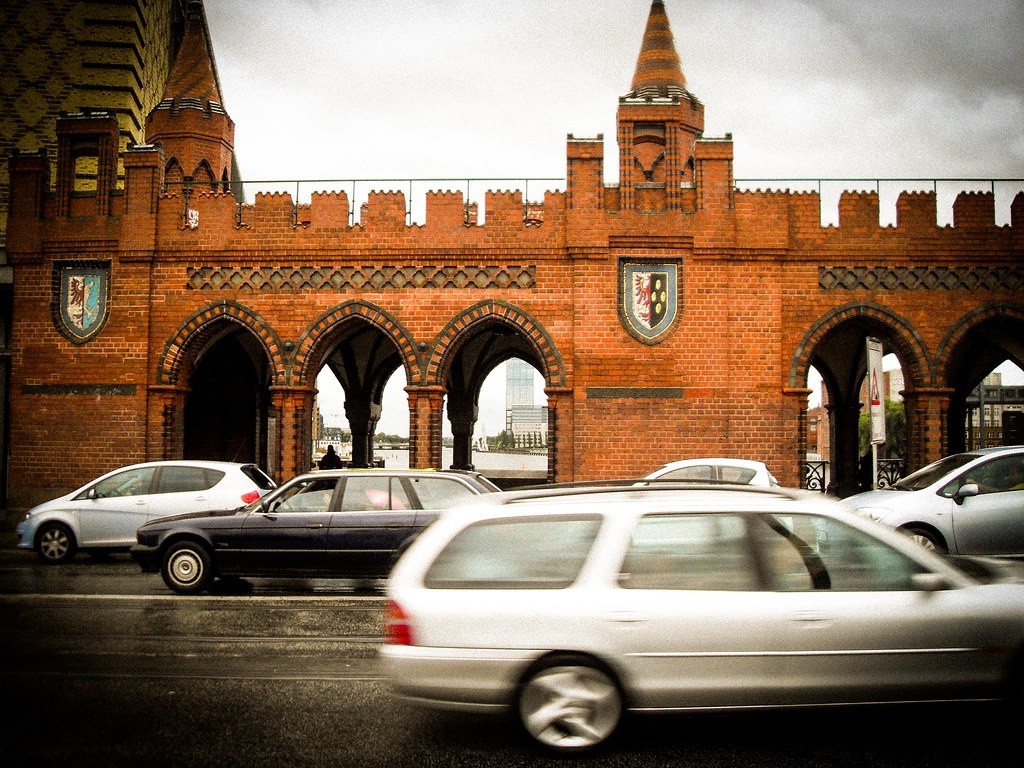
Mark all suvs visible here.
[378,479,1024,759]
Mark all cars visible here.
[815,444,1024,569]
[630,458,779,492]
[132,468,503,595]
[17,460,281,563]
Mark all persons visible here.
[966,462,1024,493]
[319,445,342,470]
